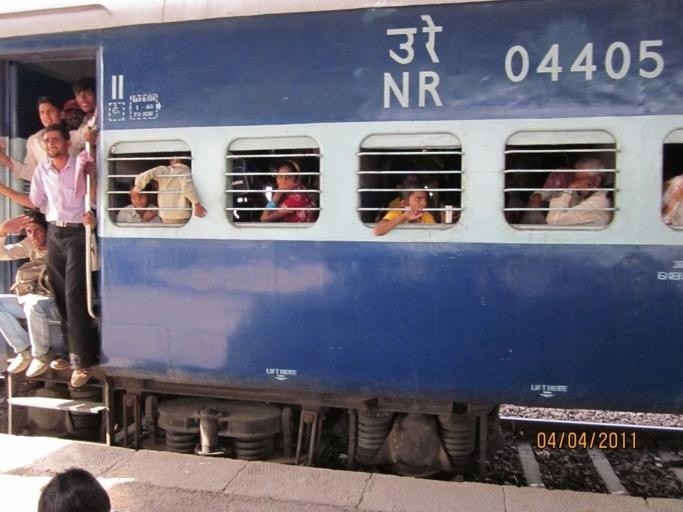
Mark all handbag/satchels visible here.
[15,261,53,297]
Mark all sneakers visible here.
[49,357,69,371]
[26,348,55,378]
[70,366,95,389]
[7,347,32,375]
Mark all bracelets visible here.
[265,201,277,211]
[563,190,576,194]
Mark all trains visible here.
[0,0,683,482]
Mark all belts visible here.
[51,221,80,228]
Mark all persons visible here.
[387,174,419,213]
[661,174,682,228]
[374,179,437,237]
[260,159,317,223]
[36,467,111,512]
[546,154,612,226]
[521,172,565,223]
[129,152,207,223]
[0,75,98,387]
[115,181,161,226]
